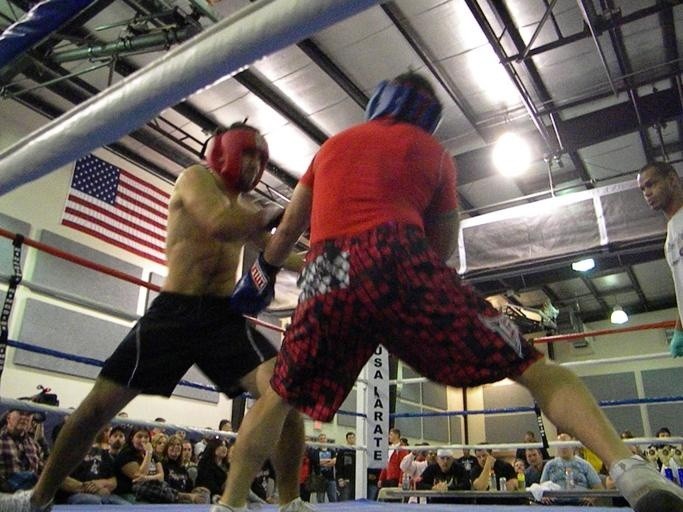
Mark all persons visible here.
[0,123,316,512]
[207,73,683,511]
[637,159,683,359]
[1,407,683,505]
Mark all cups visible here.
[500,477,506,492]
[402,472,409,490]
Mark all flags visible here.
[61,150,172,267]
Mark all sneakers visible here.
[279,498,318,512]
[1,487,55,511]
[210,503,251,512]
[612,456,683,511]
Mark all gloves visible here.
[232,253,282,316]
[671,329,683,358]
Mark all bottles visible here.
[489,472,497,490]
[517,468,525,493]
[566,469,574,489]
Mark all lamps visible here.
[610,291,629,325]
[570,258,595,272]
[487,107,532,179]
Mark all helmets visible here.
[205,129,268,196]
[365,80,443,134]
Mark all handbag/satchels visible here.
[133,478,180,502]
